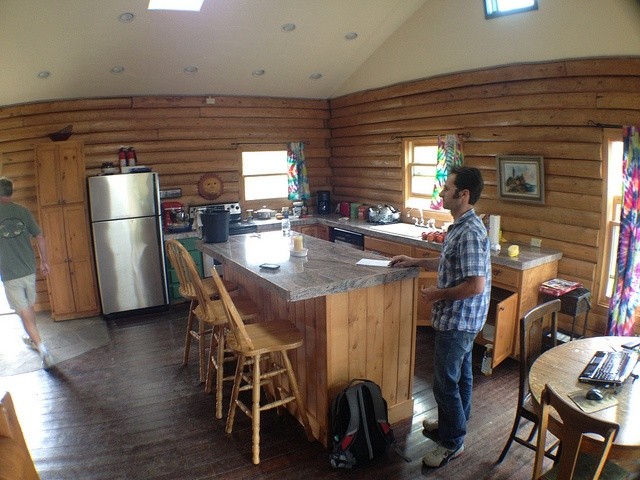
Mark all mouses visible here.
[586,389,603,400]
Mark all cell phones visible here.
[259,263,280,269]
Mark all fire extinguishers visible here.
[126,146,137,166]
[118,147,126,173]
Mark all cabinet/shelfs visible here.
[297,223,317,237]
[414,248,443,326]
[317,225,335,241]
[38,203,101,322]
[364,236,412,260]
[167,236,203,300]
[34,139,87,207]
[537,287,592,356]
[474,253,559,367]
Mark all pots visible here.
[254,206,276,219]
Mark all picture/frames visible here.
[496,155,544,206]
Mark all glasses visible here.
[443,185,458,193]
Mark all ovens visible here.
[333,227,364,250]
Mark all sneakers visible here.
[22,335,37,350]
[422,419,439,434]
[422,443,465,468]
[41,354,55,370]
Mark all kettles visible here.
[169,208,189,223]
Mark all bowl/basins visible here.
[101,167,116,174]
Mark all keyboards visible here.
[578,350,639,384]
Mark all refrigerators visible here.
[87,171,172,324]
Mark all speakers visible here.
[316,186,333,215]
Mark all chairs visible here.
[211,265,315,465]
[181,251,259,419]
[532,383,619,480]
[499,301,563,466]
[165,240,239,385]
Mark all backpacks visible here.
[328,377,411,470]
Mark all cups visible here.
[281,219,291,235]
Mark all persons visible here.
[0,179,54,370]
[389,167,493,469]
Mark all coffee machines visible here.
[315,190,331,214]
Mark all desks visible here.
[530,337,640,462]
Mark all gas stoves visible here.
[189,201,257,229]
